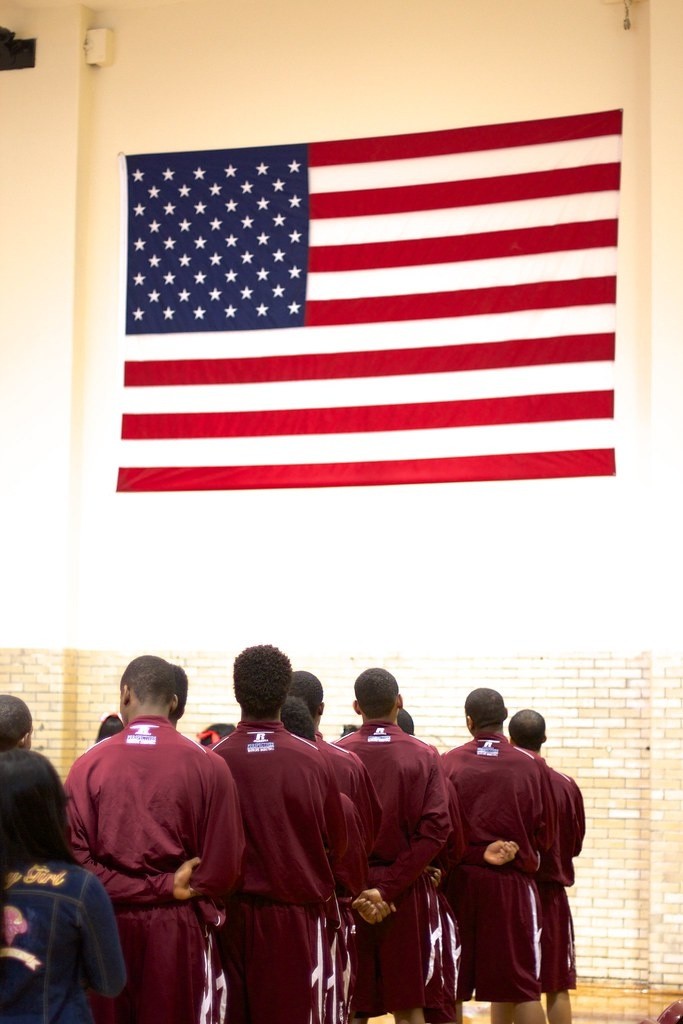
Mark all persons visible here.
[199,645,382,1024]
[440,688,547,1024]
[327,668,463,1024]
[0,694,32,753]
[491,709,585,1024]
[0,748,127,1024]
[63,656,245,1024]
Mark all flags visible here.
[113,110,623,493]
